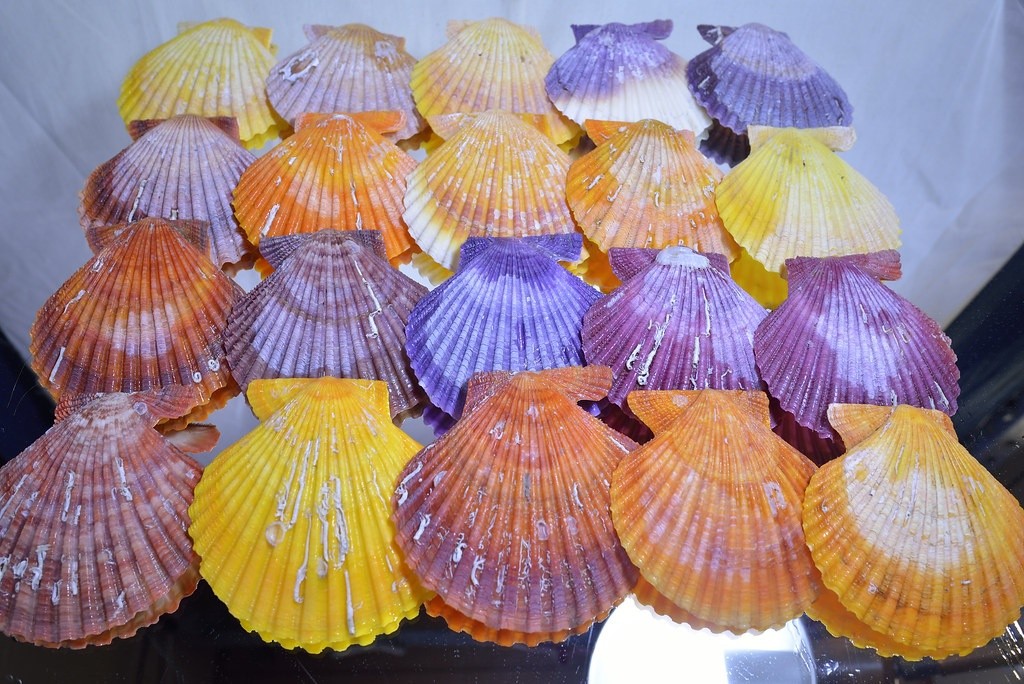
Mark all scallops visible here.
[0,18,1024,664]
